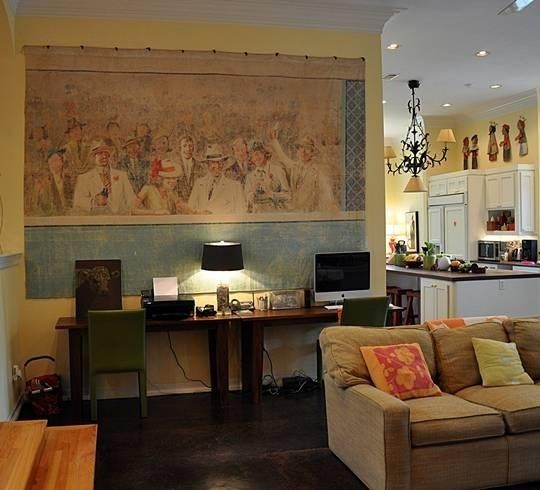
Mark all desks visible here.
[55,312,242,421]
[233,304,407,418]
[0,420,98,489]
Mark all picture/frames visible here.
[405,211,420,254]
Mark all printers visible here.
[140,289,194,321]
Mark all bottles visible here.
[489,215,507,231]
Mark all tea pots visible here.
[396,240,407,253]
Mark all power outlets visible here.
[263,343,271,356]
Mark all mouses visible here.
[389,303,394,308]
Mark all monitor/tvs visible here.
[313,250,373,305]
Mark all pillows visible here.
[318,317,540,400]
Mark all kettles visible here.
[513,247,523,260]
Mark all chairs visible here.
[316,295,392,428]
[87,309,149,420]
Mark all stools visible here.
[387,286,413,327]
[404,290,420,325]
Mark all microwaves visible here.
[477,239,507,261]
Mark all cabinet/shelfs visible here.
[424,169,484,264]
[420,277,540,325]
[484,164,536,236]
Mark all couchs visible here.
[319,316,540,490]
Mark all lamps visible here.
[384,80,456,193]
[201,241,245,314]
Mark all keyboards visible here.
[324,305,343,310]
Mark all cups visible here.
[395,254,407,267]
[422,255,436,268]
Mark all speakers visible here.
[522,239,537,263]
